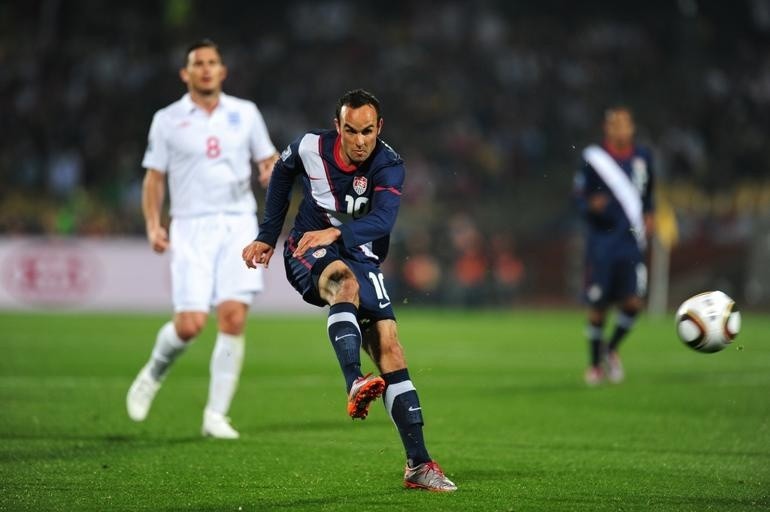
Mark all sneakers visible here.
[585,350,625,387]
[403,458,459,492]
[125,363,163,423]
[201,408,240,440]
[347,371,386,420]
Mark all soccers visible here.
[674,292,741,352]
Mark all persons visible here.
[240,87,458,491]
[123,37,281,440]
[568,103,654,385]
